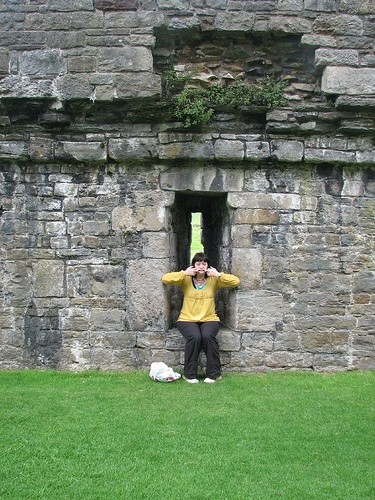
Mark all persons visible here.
[162,253,240,384]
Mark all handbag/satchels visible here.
[149,362,181,382]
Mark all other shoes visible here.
[181,374,199,383]
[204,375,223,385]
